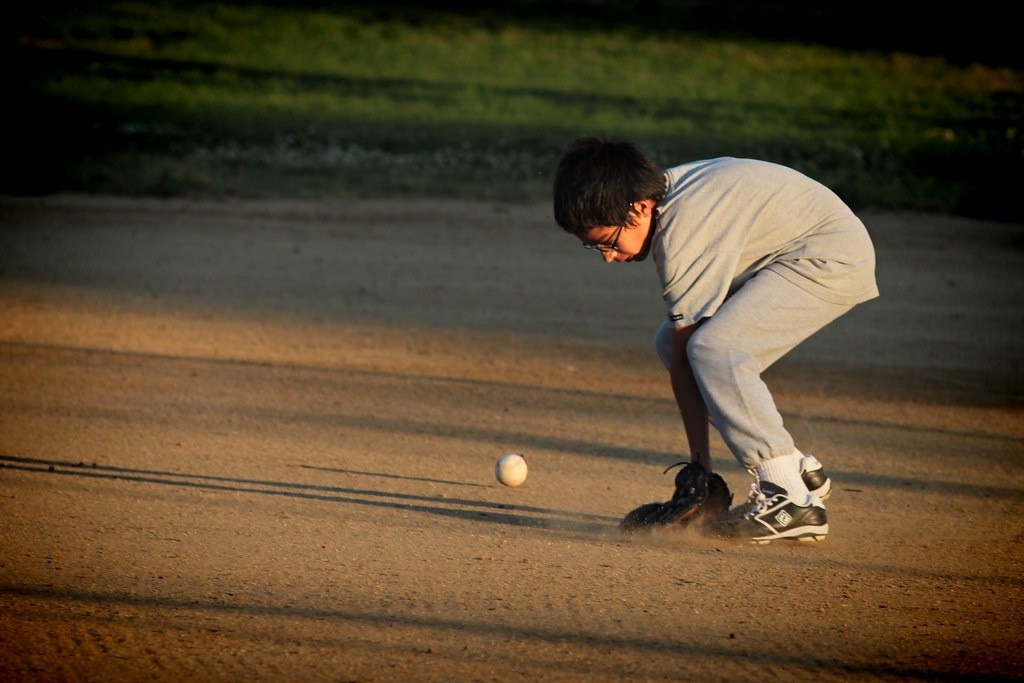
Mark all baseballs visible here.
[495,454,527,486]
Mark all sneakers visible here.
[703,454,831,544]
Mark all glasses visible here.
[583,203,633,253]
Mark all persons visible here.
[553,131,881,545]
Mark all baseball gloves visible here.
[618,462,736,535]
